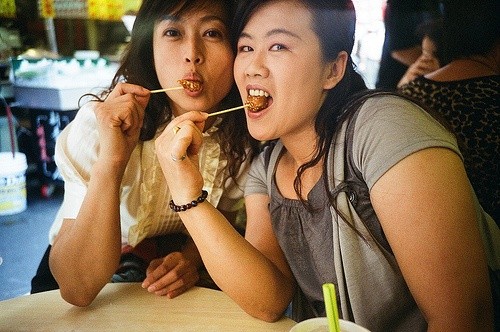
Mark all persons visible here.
[154,0,500,332]
[375,0,456,89]
[30,0,271,307]
[398,0,500,332]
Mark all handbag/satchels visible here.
[336,92,500,332]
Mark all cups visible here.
[289,317,371,332]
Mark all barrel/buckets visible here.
[0,152,27,215]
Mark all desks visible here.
[0,283,297,332]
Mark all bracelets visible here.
[169,190,208,212]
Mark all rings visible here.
[173,125,181,134]
